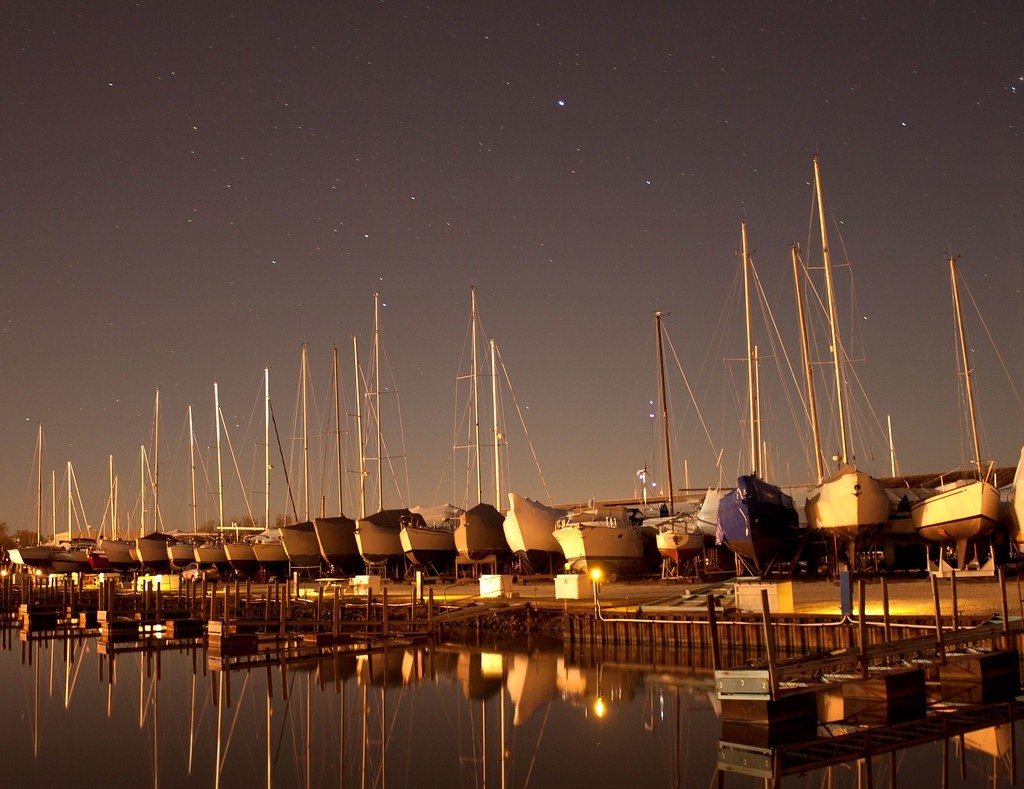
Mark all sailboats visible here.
[2,607,635,789]
[0,151,1024,576]
